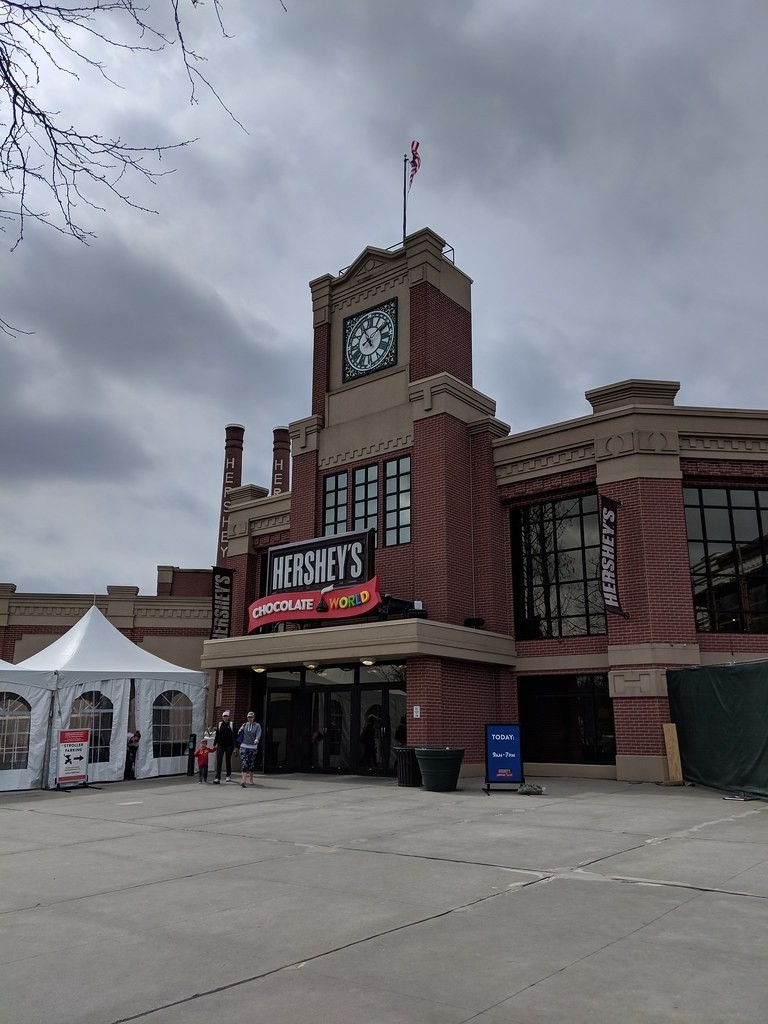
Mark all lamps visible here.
[252,666,267,673]
[360,658,377,666]
[303,662,319,669]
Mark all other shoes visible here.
[204,778,207,782]
[213,779,220,784]
[249,779,255,784]
[240,780,246,786]
[199,780,202,783]
[225,777,231,783]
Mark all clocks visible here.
[342,296,398,384]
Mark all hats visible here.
[247,712,256,718]
[222,712,229,717]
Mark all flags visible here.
[408,139,420,193]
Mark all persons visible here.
[237,711,262,788]
[194,738,217,784]
[211,709,238,784]
[359,712,380,774]
[392,713,408,778]
[125,730,142,778]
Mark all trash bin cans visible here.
[415,747,465,791]
[393,745,420,786]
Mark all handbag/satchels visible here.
[235,730,244,748]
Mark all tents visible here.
[16,604,211,789]
[0,658,59,792]
